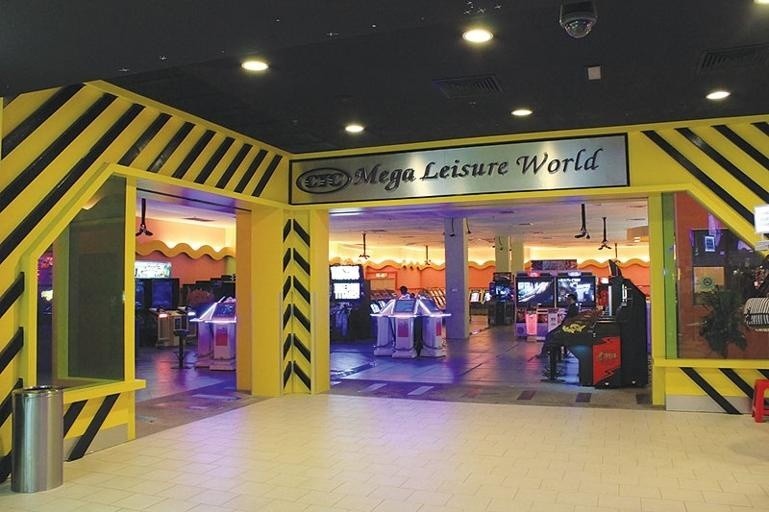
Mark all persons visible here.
[534,294,579,358]
[398,286,410,301]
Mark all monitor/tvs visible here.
[132,261,171,280]
[556,275,596,308]
[484,292,491,302]
[515,275,556,308]
[333,282,360,300]
[394,300,416,312]
[330,265,361,282]
[420,299,439,313]
[471,292,480,302]
[213,302,236,321]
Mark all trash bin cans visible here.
[11,384,63,493]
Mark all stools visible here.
[540,333,569,382]
[172,329,191,370]
[752,379,769,423]
